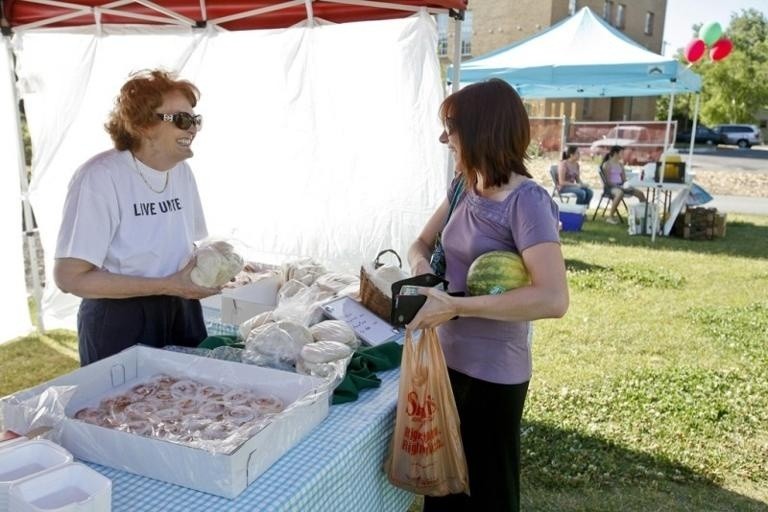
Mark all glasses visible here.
[155,112,201,130]
[443,118,457,136]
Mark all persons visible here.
[53,68,246,366]
[405,78,569,512]
[599,144,646,224]
[556,145,594,221]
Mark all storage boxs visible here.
[655,161,686,183]
[1,342,328,500]
[556,202,588,232]
[221,268,284,325]
[0,439,74,486]
[8,461,112,512]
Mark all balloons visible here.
[684,38,706,65]
[698,21,723,49]
[708,39,733,61]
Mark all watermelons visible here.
[466,251,531,297]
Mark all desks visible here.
[0,316,417,512]
[623,174,696,236]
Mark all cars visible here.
[676,125,728,148]
[589,123,650,157]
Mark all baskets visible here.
[360,250,401,322]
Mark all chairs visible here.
[592,165,628,224]
[549,165,577,203]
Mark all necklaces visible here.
[131,150,169,194]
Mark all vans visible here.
[711,124,761,149]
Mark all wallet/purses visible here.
[392,274,465,323]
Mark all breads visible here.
[191,242,360,374]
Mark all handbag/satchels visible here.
[430,176,464,273]
